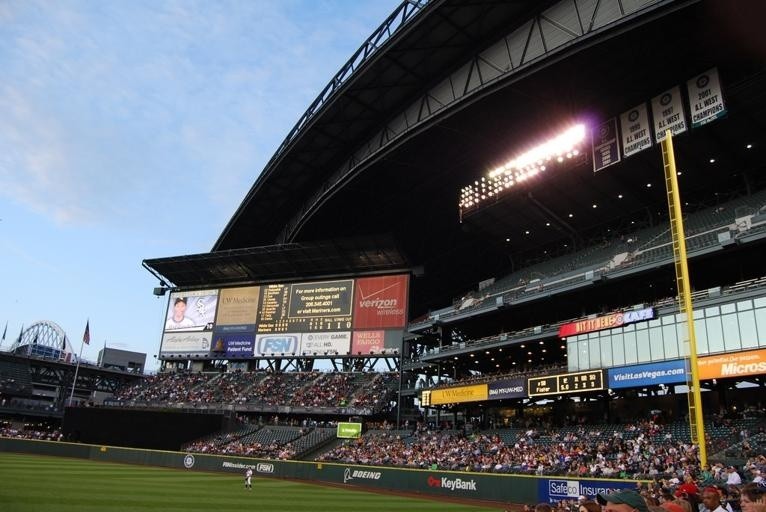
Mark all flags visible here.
[32,334,37,343]
[83,321,91,345]
[62,336,66,350]
[17,328,23,342]
[2,326,8,339]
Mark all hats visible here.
[660,502,687,512]
[702,487,717,492]
[675,482,698,497]
[597,491,649,512]
[752,476,766,485]
[727,466,734,469]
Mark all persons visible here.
[521,398,766,511]
[166,296,197,328]
[315,414,523,473]
[0,415,64,441]
[116,368,391,461]
[244,466,252,491]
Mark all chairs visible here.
[434,193,766,318]
[179,418,766,483]
